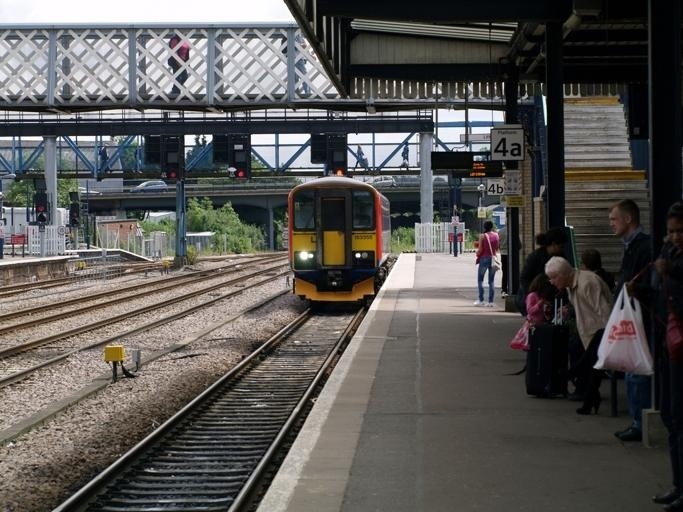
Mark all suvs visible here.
[129,179,168,193]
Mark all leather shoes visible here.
[615,425,642,442]
[653,487,682,511]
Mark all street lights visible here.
[477,184,485,234]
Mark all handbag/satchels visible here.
[510,320,529,351]
[666,308,683,362]
[491,255,500,270]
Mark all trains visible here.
[286,174,391,311]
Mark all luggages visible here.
[525,294,570,396]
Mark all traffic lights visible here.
[68,203,78,218]
[331,150,347,176]
[231,149,248,178]
[33,191,47,212]
[164,142,179,179]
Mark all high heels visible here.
[576,398,600,414]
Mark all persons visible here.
[134,146,142,174]
[517,227,613,415]
[354,145,367,171]
[98,144,112,174]
[607,198,657,443]
[472,219,499,307]
[281,28,316,99]
[651,199,682,511]
[400,141,410,172]
[167,32,192,101]
[498,218,507,299]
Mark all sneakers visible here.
[473,299,494,307]
[502,291,506,298]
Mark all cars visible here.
[70,187,101,196]
